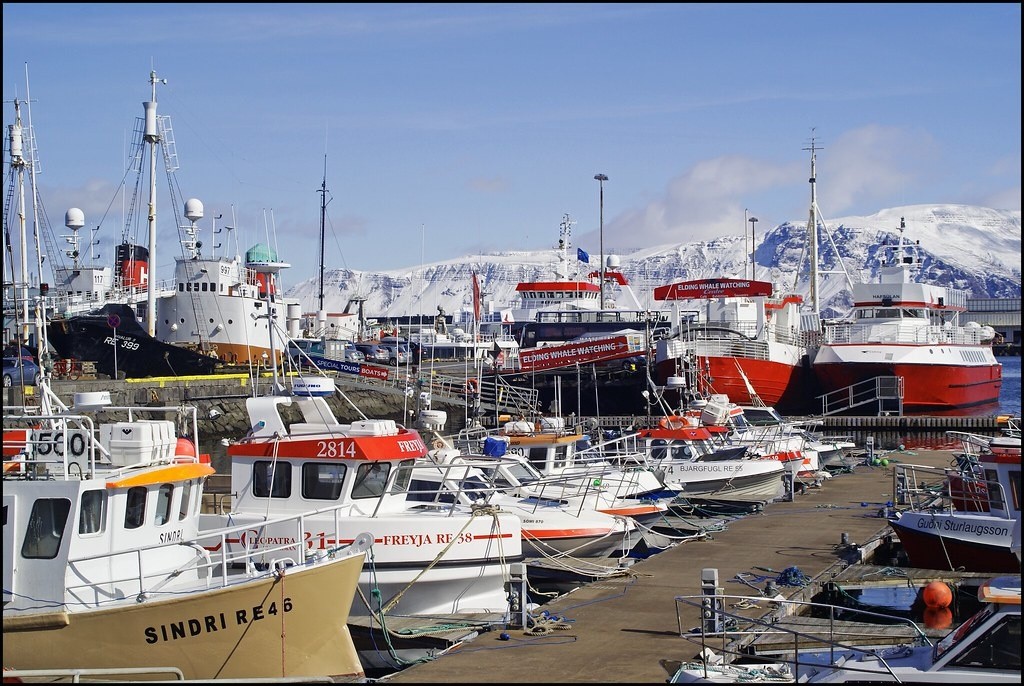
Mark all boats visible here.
[674,420,1021,683]
[2,63,857,683]
[812,216,1004,414]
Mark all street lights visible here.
[748,217,758,282]
[594,173,608,320]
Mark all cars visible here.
[355,339,428,367]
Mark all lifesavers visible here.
[465,378,479,393]
[659,416,688,430]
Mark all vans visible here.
[284,338,365,363]
